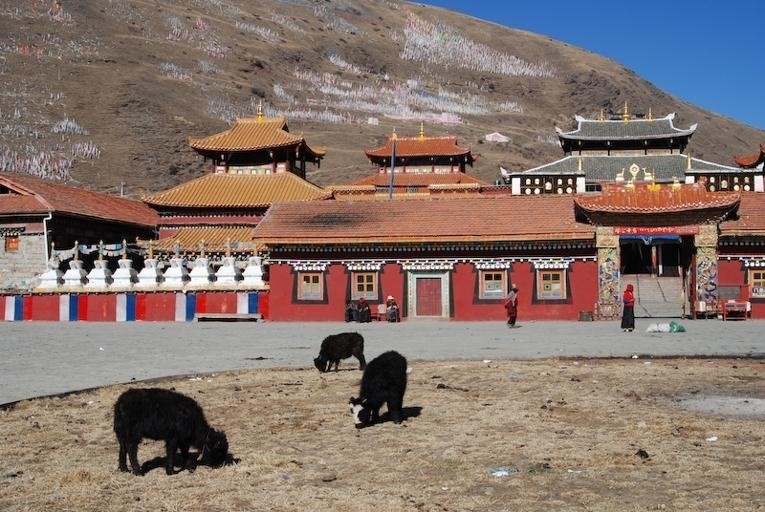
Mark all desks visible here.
[724,300,747,321]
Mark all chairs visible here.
[694,301,707,320]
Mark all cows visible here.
[348,350,407,429]
[113,386,229,476]
[312,332,366,373]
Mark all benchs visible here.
[368,304,386,320]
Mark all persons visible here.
[344,300,357,322]
[386,295,398,322]
[504,287,519,328]
[620,283,635,332]
[357,297,369,323]
